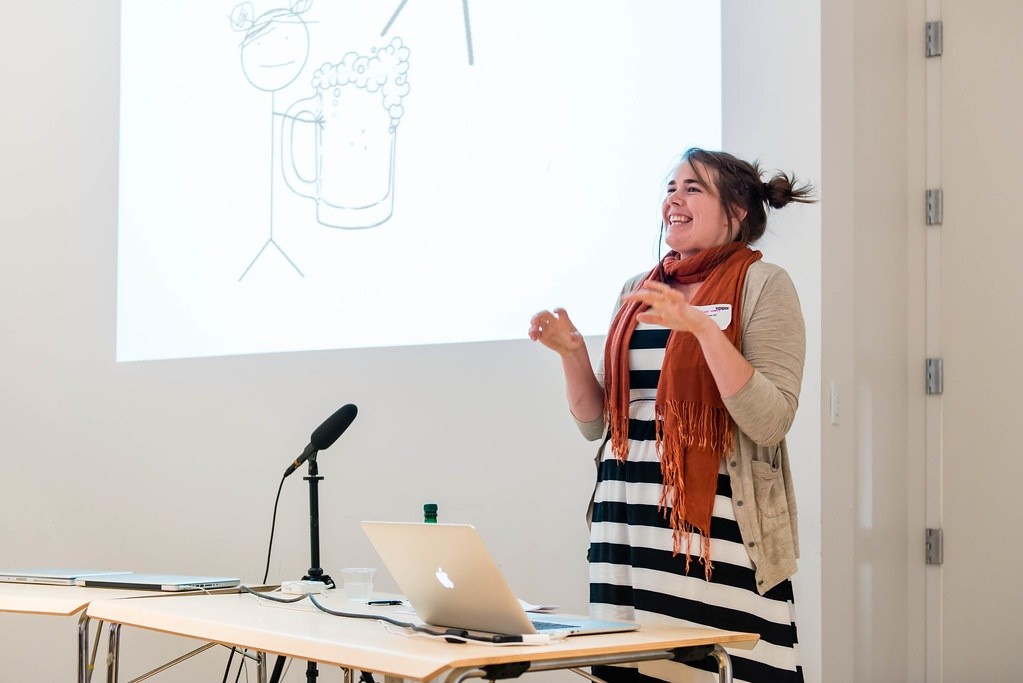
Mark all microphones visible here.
[281,403,359,476]
[657,216,677,290]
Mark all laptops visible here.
[75,574,240,592]
[360,520,641,640]
[0,569,134,586]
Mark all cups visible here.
[342,567,375,601]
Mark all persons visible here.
[527,146,815,683]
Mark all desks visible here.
[0,570,760,683]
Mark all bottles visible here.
[424,504,438,523]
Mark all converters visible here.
[280,579,323,596]
